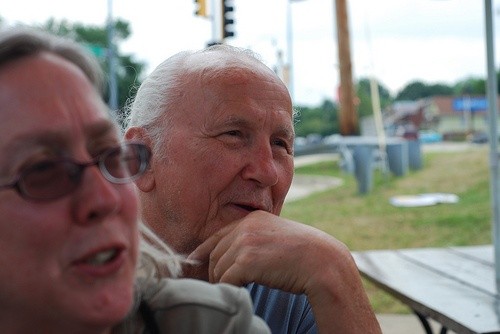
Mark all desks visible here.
[350,244,500,334]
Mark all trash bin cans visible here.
[346,142,380,196]
[407,141,422,172]
[385,143,404,177]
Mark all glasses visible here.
[0,143,151,203]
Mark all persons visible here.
[125,45,383,334]
[0,25,270,334]
